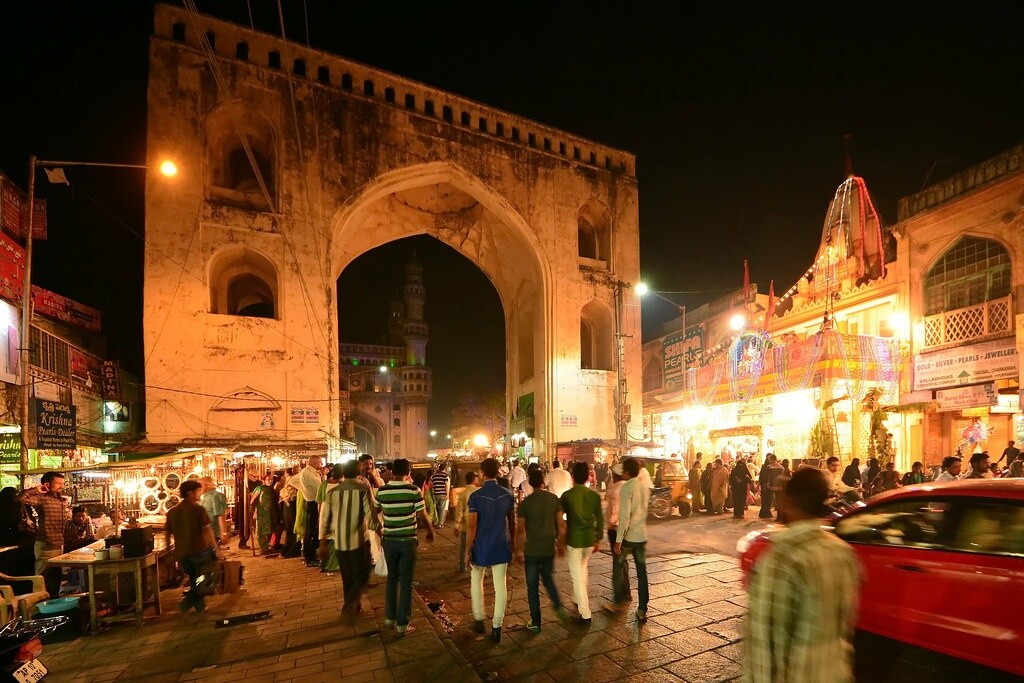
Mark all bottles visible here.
[110,545,123,559]
[95,549,110,561]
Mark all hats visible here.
[612,464,623,475]
[466,471,479,482]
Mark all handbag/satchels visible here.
[23,507,36,533]
[374,546,388,576]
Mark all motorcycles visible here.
[615,454,692,519]
[0,615,71,683]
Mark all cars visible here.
[732,475,1024,683]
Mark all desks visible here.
[88,550,162,639]
[46,530,176,626]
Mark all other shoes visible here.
[193,605,208,616]
[491,626,501,643]
[385,619,396,629]
[602,602,630,614]
[571,616,591,625]
[527,621,541,634]
[238,542,250,549]
[396,625,416,637]
[625,593,633,600]
[636,609,647,622]
[473,621,485,635]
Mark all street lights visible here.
[347,367,388,414]
[20,154,179,493]
[636,281,688,414]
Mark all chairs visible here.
[0,572,51,630]
[970,519,1007,549]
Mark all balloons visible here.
[962,420,989,442]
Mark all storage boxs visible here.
[30,608,83,645]
[449,487,467,508]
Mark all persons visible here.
[164,476,228,617]
[231,454,451,638]
[0,471,99,599]
[454,454,654,643]
[743,467,859,682]
[671,440,1024,522]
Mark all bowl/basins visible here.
[36,597,80,614]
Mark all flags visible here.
[764,283,776,327]
[743,260,750,300]
